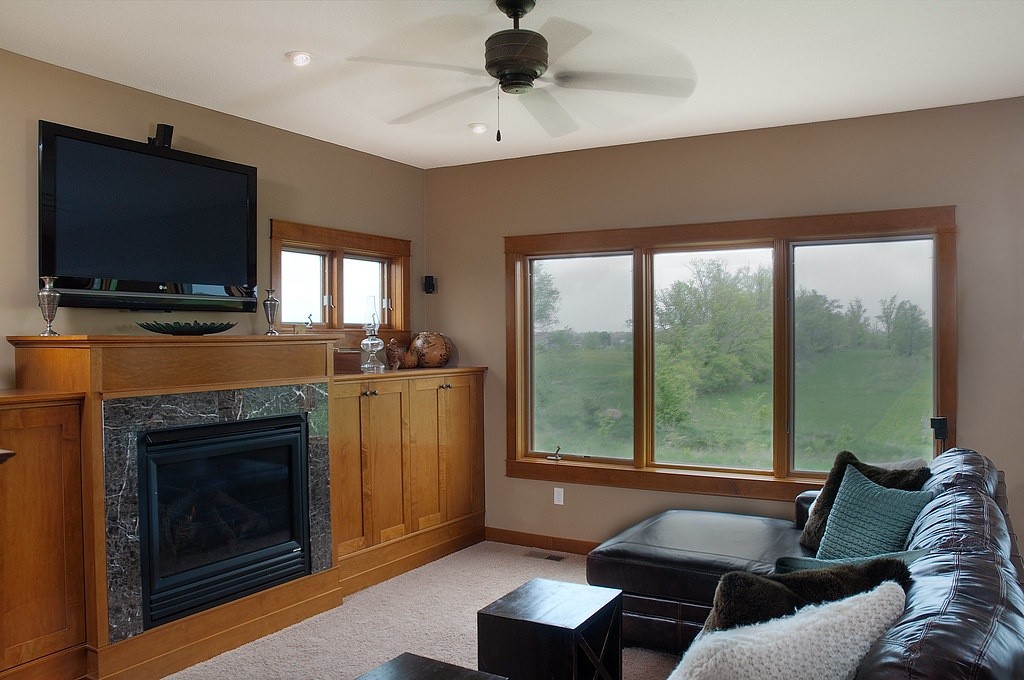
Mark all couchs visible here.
[586,448,1024,680]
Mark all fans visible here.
[346,0,697,142]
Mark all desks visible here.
[478,577,623,680]
[356,652,508,680]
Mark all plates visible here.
[135,320,238,335]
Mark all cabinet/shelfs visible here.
[0,389,86,680]
[335,367,487,596]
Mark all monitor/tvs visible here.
[38,121,257,313]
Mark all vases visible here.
[36,275,61,336]
[411,331,452,369]
[263,288,279,336]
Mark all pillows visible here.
[816,464,934,559]
[800,451,932,551]
[808,458,927,517]
[701,557,912,634]
[668,580,907,679]
[775,547,932,574]
